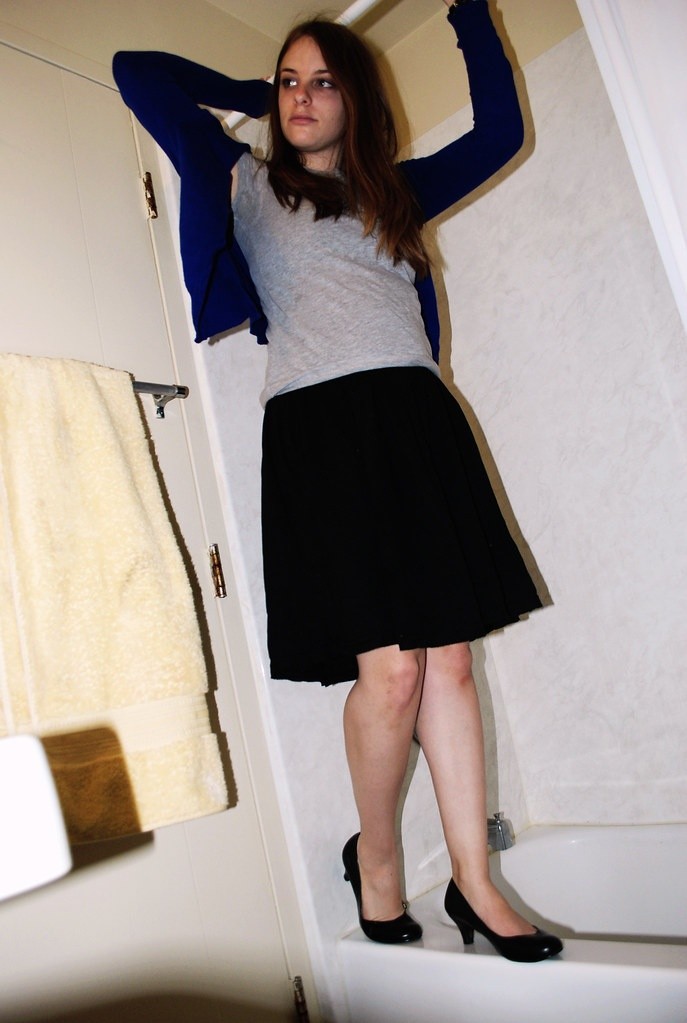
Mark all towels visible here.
[0,349,235,908]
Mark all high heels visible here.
[444,875,563,962]
[342,833,423,944]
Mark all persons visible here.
[113,0,565,963]
